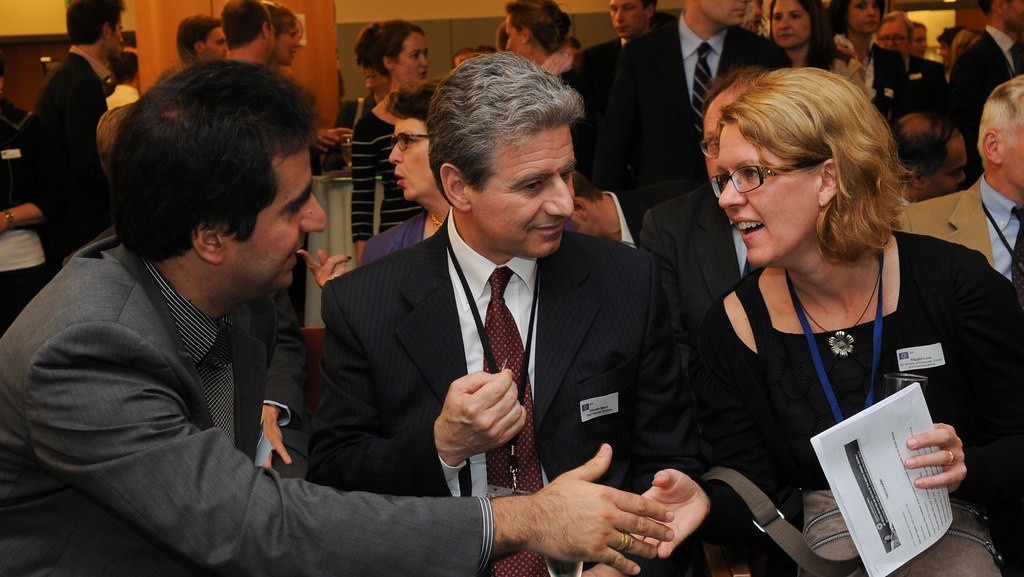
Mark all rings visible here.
[948,449,955,465]
[617,531,630,550]
[317,135,323,142]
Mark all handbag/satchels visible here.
[801,491,1000,577]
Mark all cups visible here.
[879,373,929,405]
[341,134,352,167]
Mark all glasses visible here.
[876,34,908,42]
[710,159,824,197]
[390,133,428,151]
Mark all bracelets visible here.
[2,209,15,230]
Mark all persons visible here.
[0,0,1024,378]
[306,55,714,577]
[0,62,675,577]
[626,70,1024,577]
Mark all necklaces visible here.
[784,249,885,359]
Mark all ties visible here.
[692,42,713,141]
[481,267,549,576]
[1012,206,1024,310]
